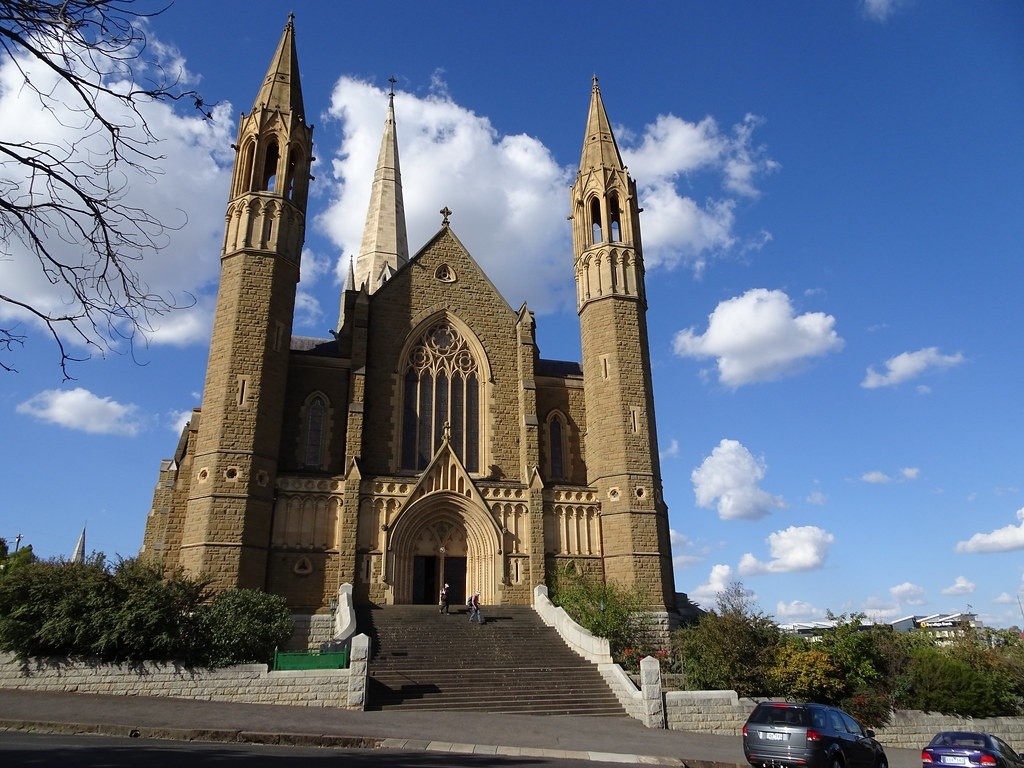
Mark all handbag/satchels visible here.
[442,595,449,600]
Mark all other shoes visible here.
[440,609,444,614]
[446,613,449,615]
[477,622,483,623]
[468,621,472,623]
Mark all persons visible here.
[468,591,483,624]
[439,583,452,615]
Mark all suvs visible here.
[742,703,889,768]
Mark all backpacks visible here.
[468,596,472,607]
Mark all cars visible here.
[921,732,1024,768]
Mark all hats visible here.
[475,590,481,595]
[445,583,449,587]
